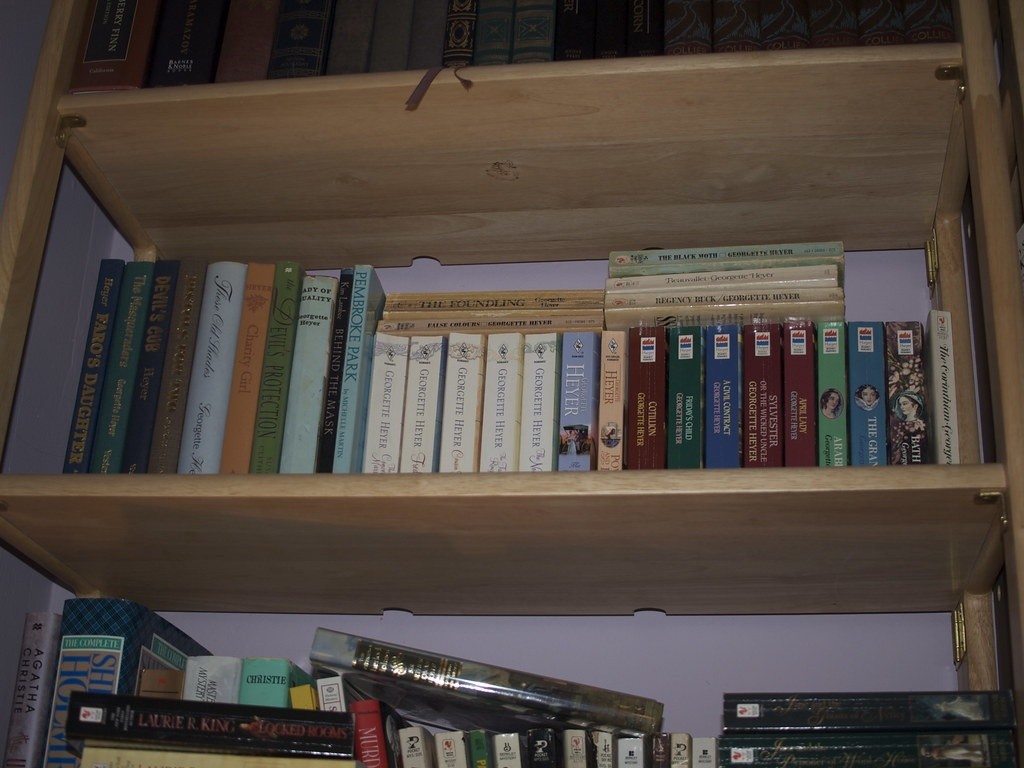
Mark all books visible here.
[69,0,959,93]
[4,598,1017,768]
[61,241,960,473]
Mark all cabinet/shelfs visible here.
[0,0,1024,767]
